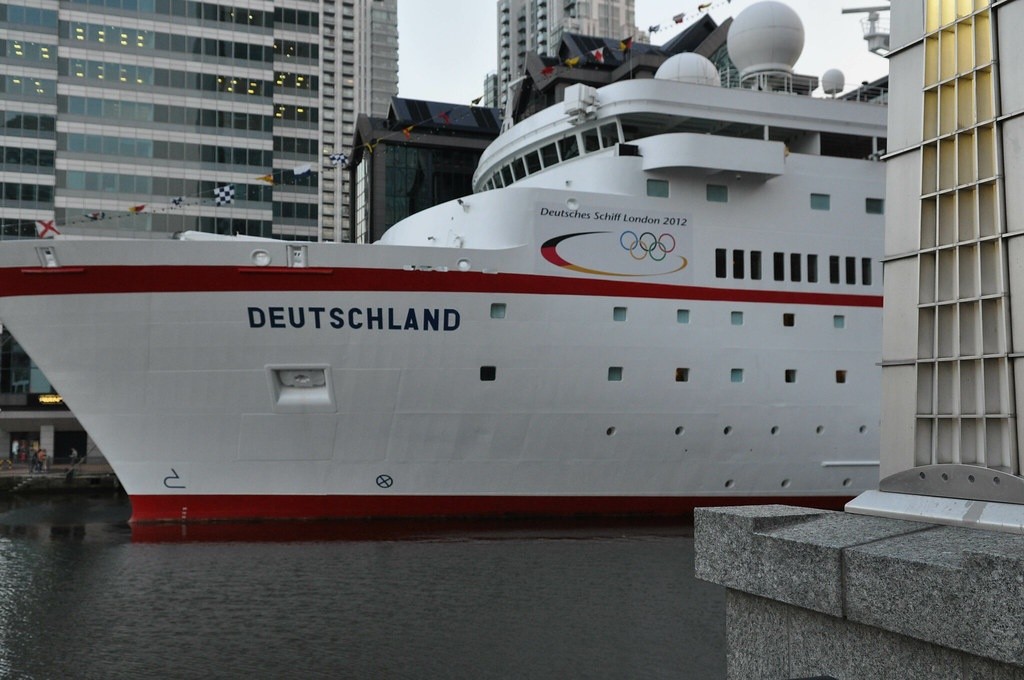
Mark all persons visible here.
[12,439,27,464]
[27,448,79,474]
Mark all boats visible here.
[0,0,886,522]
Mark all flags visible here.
[293,165,312,179]
[170,196,186,205]
[437,109,455,126]
[619,37,633,50]
[257,176,280,186]
[129,204,145,214]
[329,152,350,166]
[85,212,106,220]
[590,46,605,64]
[212,184,236,207]
[402,125,416,140]
[647,22,660,32]
[34,220,60,238]
[673,12,685,24]
[540,66,554,78]
[470,97,482,108]
[364,138,381,153]
[697,3,712,12]
[567,56,580,69]
